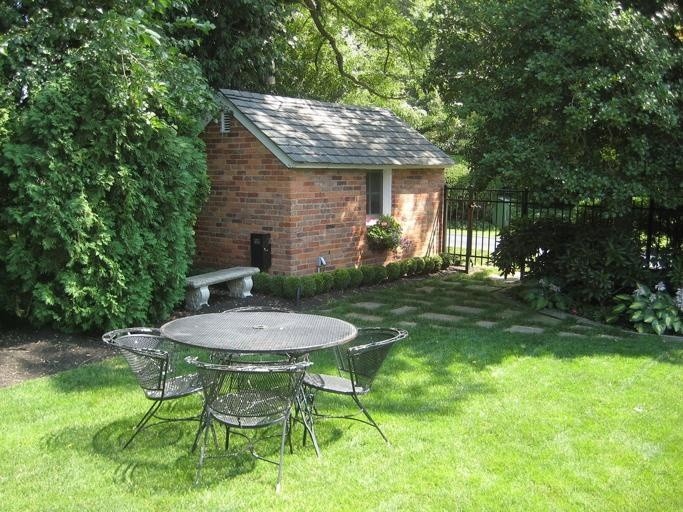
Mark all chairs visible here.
[182,351,319,493]
[295,326,411,448]
[100,324,224,451]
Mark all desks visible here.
[159,310,358,455]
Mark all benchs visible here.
[182,264,261,312]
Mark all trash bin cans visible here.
[250,233,271,270]
